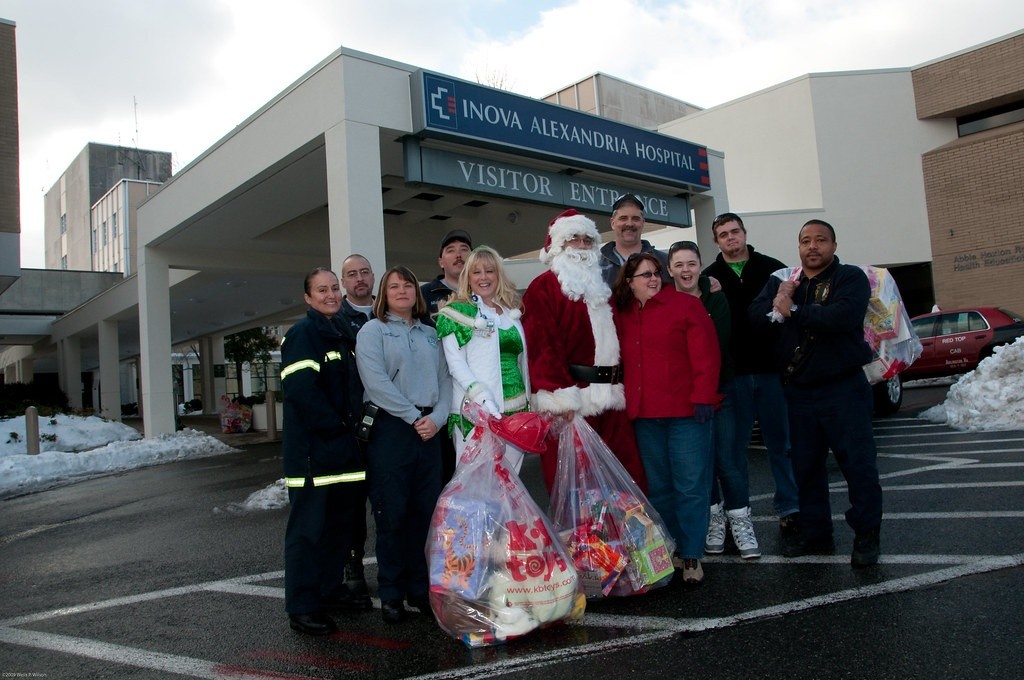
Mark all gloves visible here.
[694,405,714,425]
[468,381,502,419]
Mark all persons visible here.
[436,243,532,476]
[520,209,649,499]
[701,213,803,537]
[609,253,726,582]
[748,220,884,567]
[355,265,453,623]
[280,266,365,634]
[338,228,473,609]
[599,192,761,559]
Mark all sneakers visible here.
[726,506,763,559]
[784,534,832,556]
[780,513,801,534]
[681,558,705,585]
[703,502,726,554]
[407,593,434,615]
[851,530,879,567]
[381,600,408,624]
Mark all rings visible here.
[423,434,427,437]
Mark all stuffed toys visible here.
[488,562,577,624]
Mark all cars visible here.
[896,304,1024,382]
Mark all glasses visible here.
[344,269,372,278]
[567,236,595,246]
[712,213,735,230]
[632,270,661,278]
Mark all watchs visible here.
[789,304,798,317]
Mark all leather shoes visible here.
[289,611,336,633]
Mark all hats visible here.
[439,228,473,249]
[613,193,645,210]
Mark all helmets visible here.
[488,412,548,455]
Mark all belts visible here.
[568,364,624,386]
